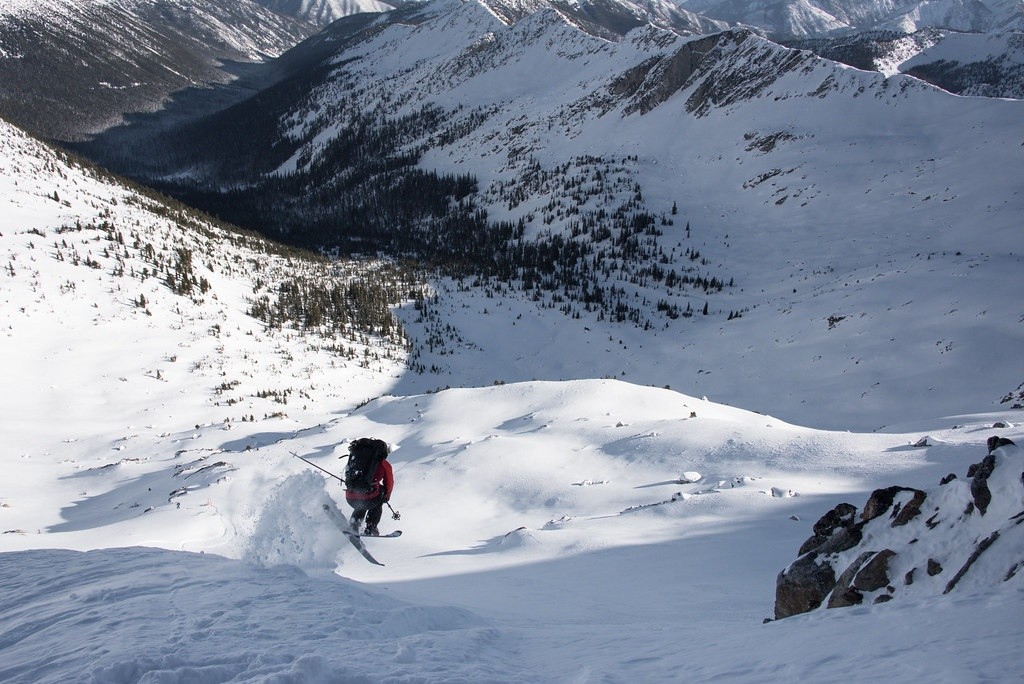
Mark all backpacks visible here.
[345,438,383,490]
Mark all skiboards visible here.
[322,502,403,566]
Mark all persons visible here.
[344,438,394,536]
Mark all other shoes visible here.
[363,523,379,535]
[349,524,360,536]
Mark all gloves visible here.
[382,497,389,503]
